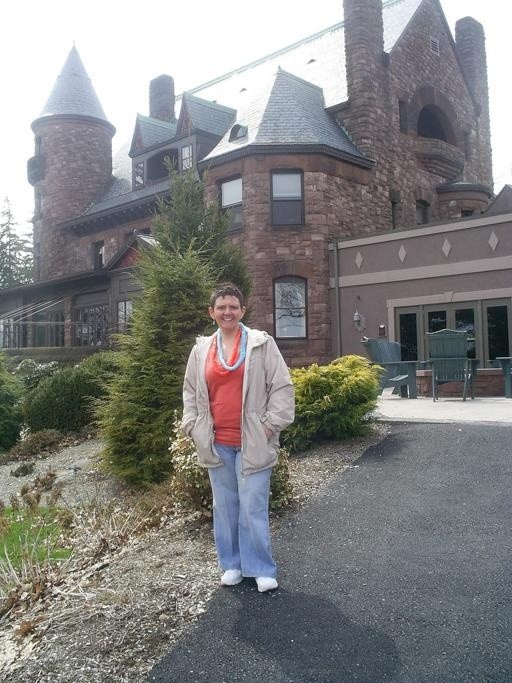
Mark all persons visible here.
[182,285,295,595]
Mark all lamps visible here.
[353,308,365,332]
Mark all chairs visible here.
[362,326,511,399]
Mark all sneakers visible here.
[254,577,278,592]
[221,570,243,586]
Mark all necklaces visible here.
[216,322,246,371]
